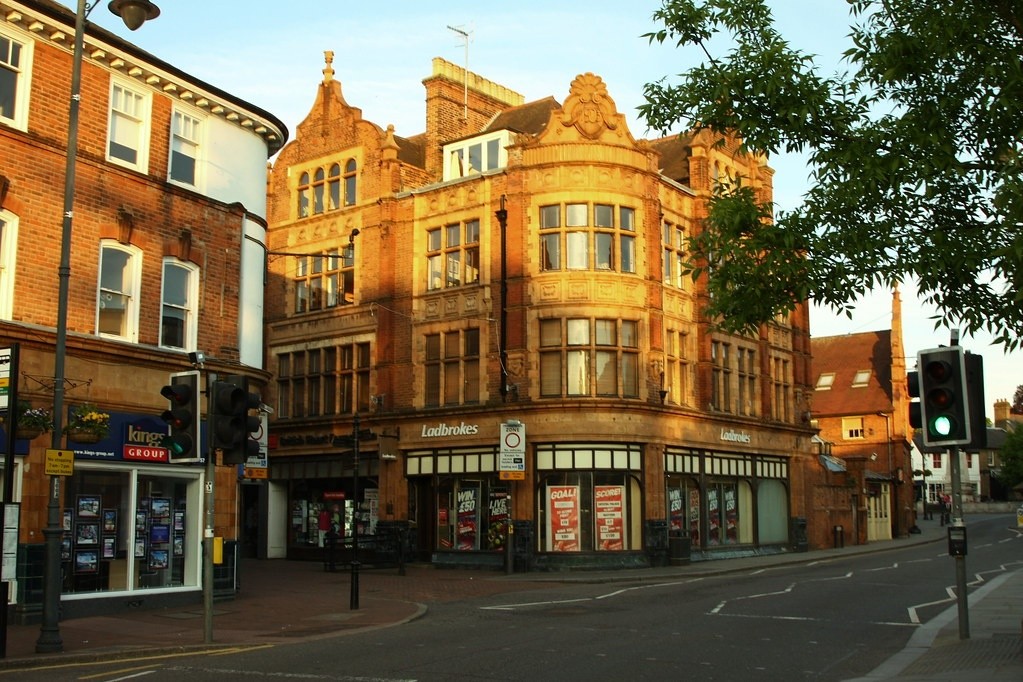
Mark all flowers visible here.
[0,404,54,434]
[63,404,115,439]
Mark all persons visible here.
[938,493,952,512]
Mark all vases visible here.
[69,428,101,443]
[3,424,41,439]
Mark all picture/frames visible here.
[61,494,117,574]
[134,496,186,571]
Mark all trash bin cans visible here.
[669,528,691,565]
[834,525,844,548]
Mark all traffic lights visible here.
[160,372,200,464]
[211,382,245,447]
[223,379,260,464]
[917,347,972,447]
